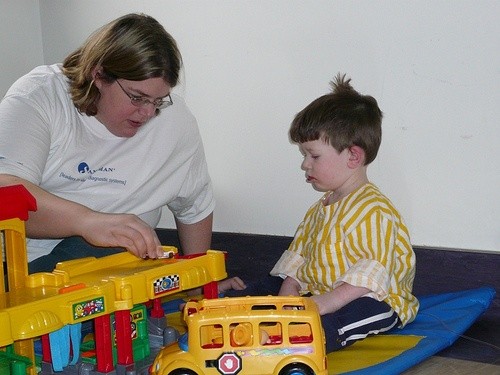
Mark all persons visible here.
[270,72,419,353]
[0,11,250,341]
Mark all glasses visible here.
[115,78,174,109]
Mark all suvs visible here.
[148,295,327,375]
